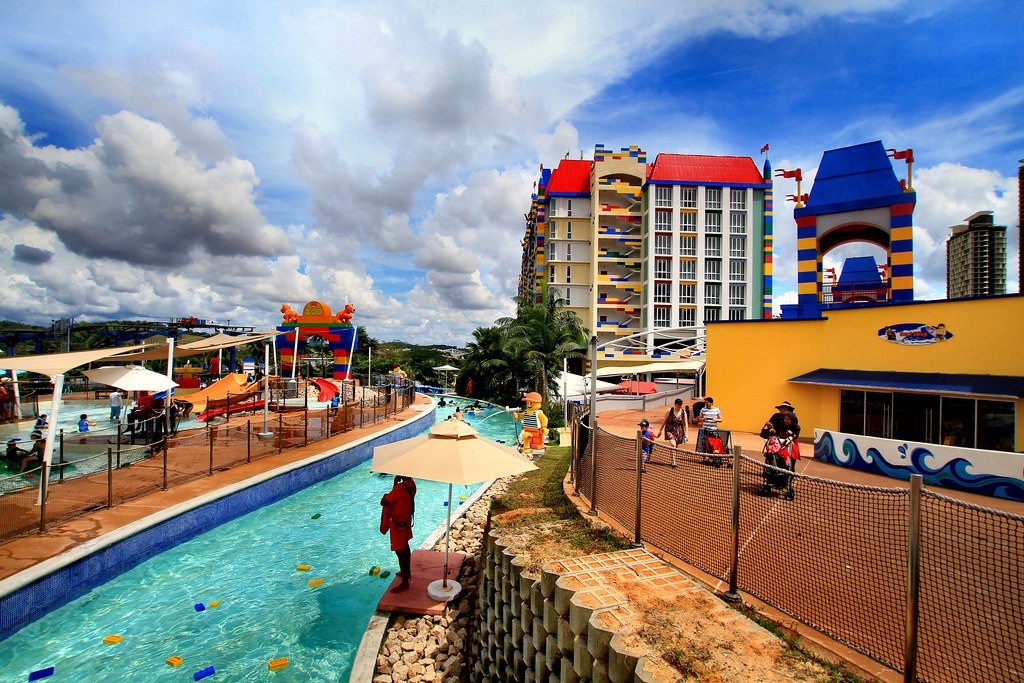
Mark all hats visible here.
[30,431,42,436]
[675,398,683,405]
[638,421,650,426]
[776,400,794,412]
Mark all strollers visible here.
[760,426,802,502]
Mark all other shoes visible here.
[395,572,413,579]
[390,580,410,594]
[671,463,679,468]
[646,459,652,463]
[641,469,647,473]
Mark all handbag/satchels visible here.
[760,422,772,440]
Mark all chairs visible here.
[130,398,164,445]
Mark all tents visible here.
[0,326,302,505]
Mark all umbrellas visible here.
[372,417,540,601]
[81,365,179,444]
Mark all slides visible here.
[307,377,341,402]
[172,372,281,413]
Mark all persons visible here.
[109,388,123,419]
[301,368,306,381]
[646,425,662,462]
[78,414,96,433]
[766,400,801,472]
[123,391,156,433]
[438,397,483,420]
[658,398,688,467]
[699,397,723,431]
[18,430,47,474]
[513,392,548,449]
[0,376,13,425]
[637,420,651,473]
[5,441,27,468]
[33,414,49,430]
[379,476,416,593]
[330,392,340,416]
[247,373,253,382]
